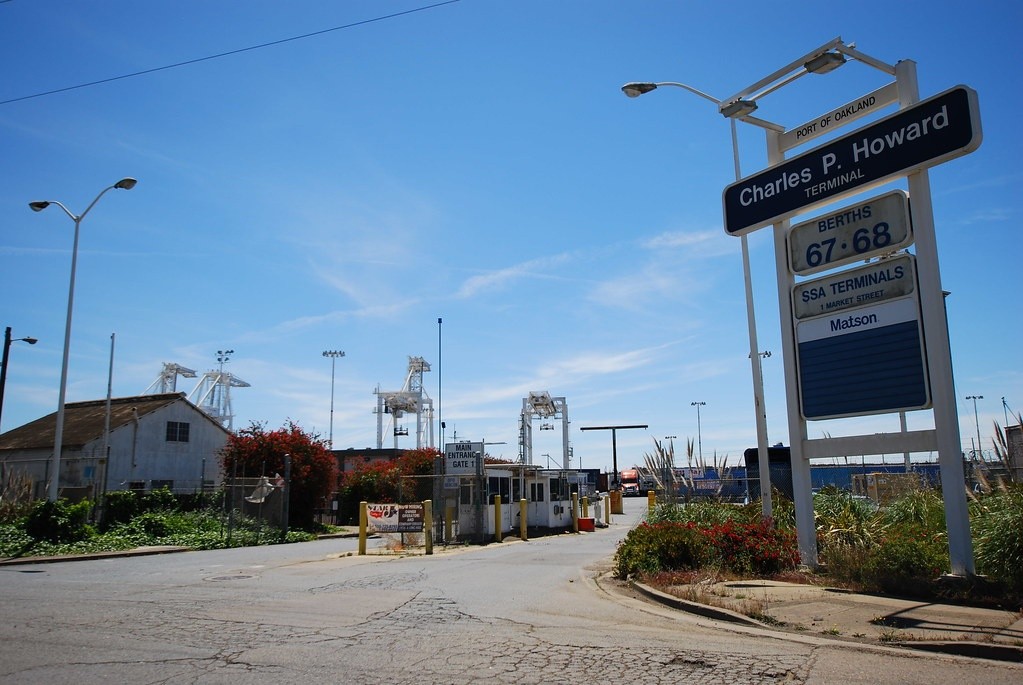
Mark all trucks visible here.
[618,470,641,497]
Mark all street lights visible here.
[214,349,235,414]
[665,436,677,466]
[437,317,443,452]
[441,422,446,454]
[0,327,38,421]
[28,176,138,503]
[966,395,984,454]
[690,401,707,467]
[621,80,772,516]
[322,350,346,448]
